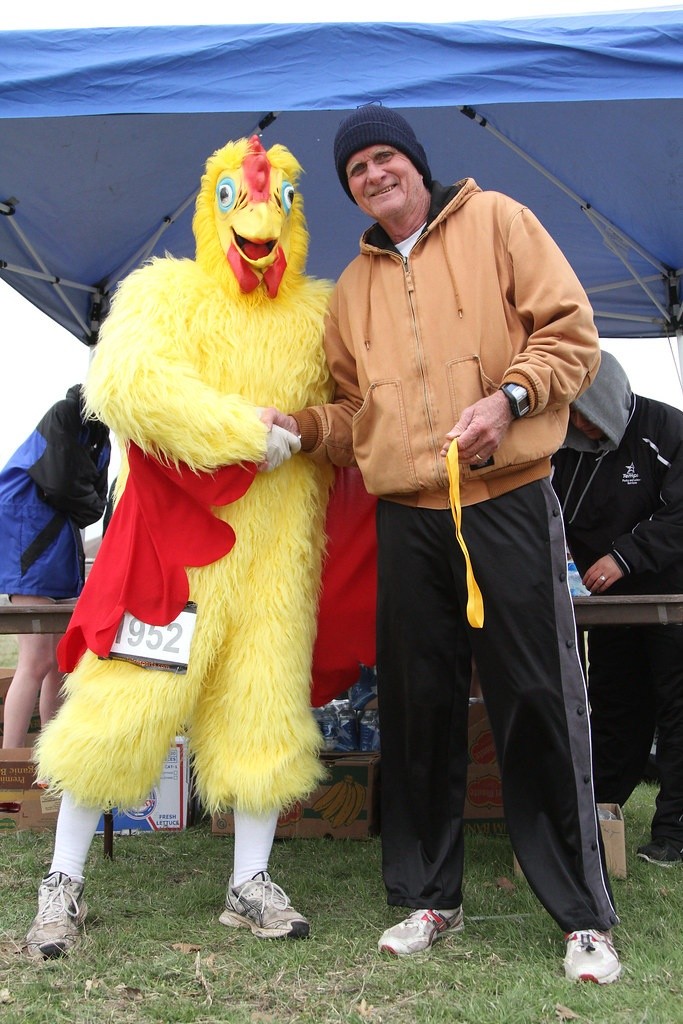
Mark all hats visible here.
[333,105,432,207]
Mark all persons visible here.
[251,101,626,990]
[26,128,343,965]
[548,345,683,869]
[0,380,116,753]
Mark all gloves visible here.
[262,423,302,473]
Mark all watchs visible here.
[499,382,531,419]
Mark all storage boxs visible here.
[513,804,627,878]
[96,737,191,831]
[211,754,380,842]
[0,745,60,827]
[0,667,43,734]
[461,695,503,820]
[348,677,379,709]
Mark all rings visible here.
[475,453,483,460]
[598,575,606,581]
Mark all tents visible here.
[0,0,683,363]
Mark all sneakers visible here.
[378,902,465,960]
[218,870,310,942]
[561,927,621,988]
[25,869,89,962]
[635,838,682,868]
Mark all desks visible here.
[1,595,682,625]
